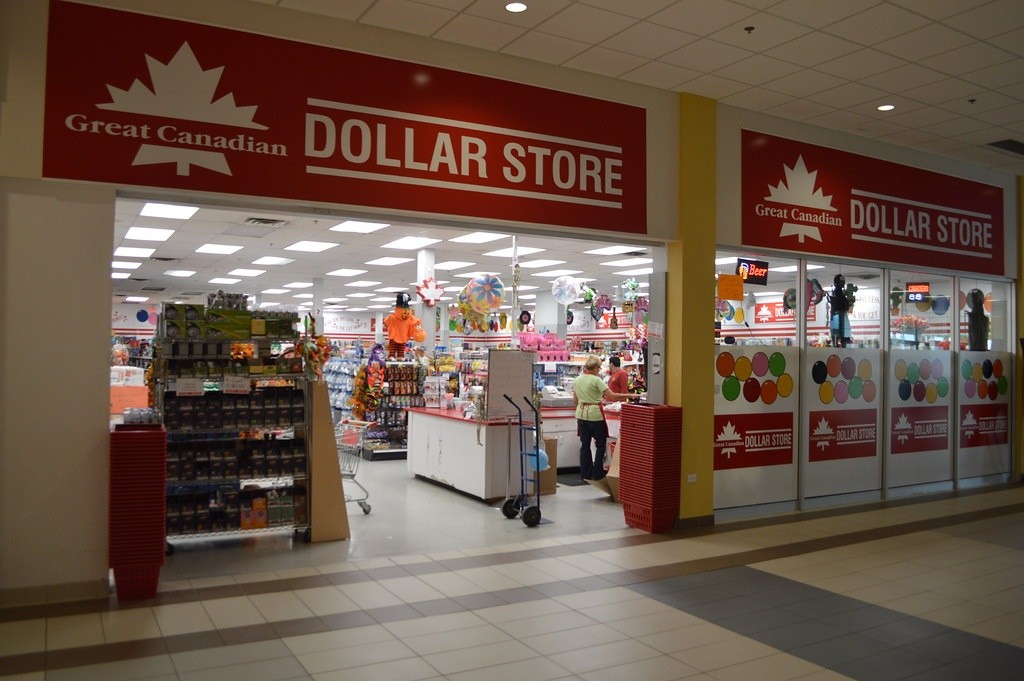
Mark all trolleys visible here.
[265,419,378,515]
[502,394,554,527]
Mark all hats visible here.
[406,344,410,347]
[392,294,413,307]
[418,346,427,353]
[413,347,418,351]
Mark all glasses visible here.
[597,366,603,370]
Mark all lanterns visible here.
[415,329,426,342]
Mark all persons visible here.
[573,353,640,480]
[382,294,420,361]
[450,305,456,319]
[605,356,627,402]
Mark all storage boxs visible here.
[532,435,558,496]
[163,302,251,341]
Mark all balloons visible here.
[623,279,639,301]
[552,276,579,305]
[466,274,505,314]
[532,449,548,471]
[458,283,484,322]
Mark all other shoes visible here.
[587,475,592,480]
[593,470,607,480]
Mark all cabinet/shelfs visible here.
[162,355,312,544]
